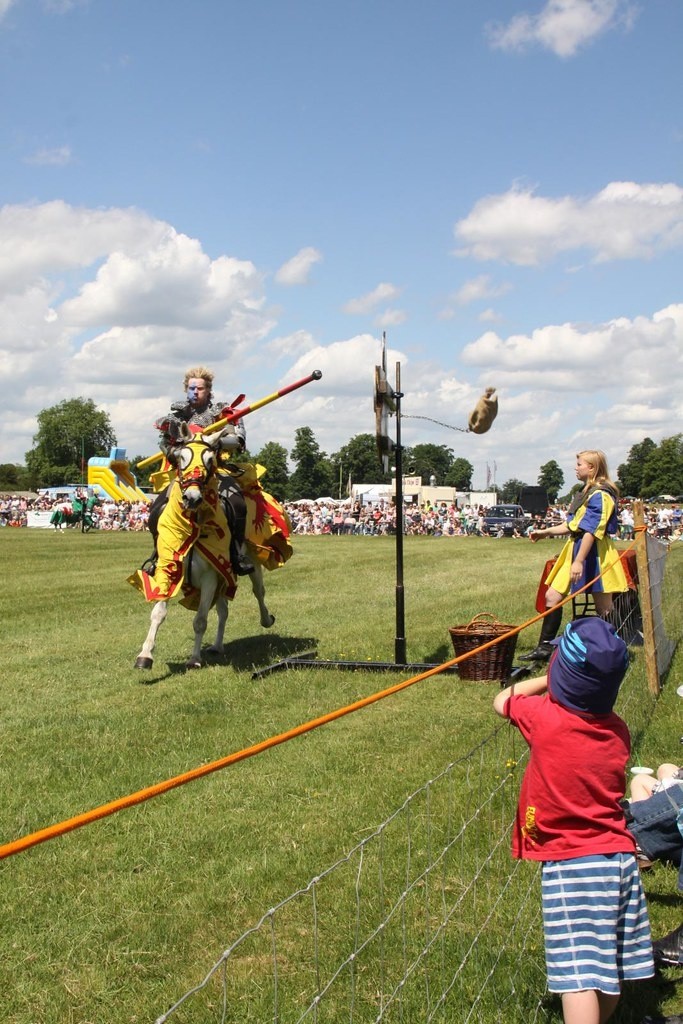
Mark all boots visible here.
[148,559,160,575]
[517,606,562,661]
[233,540,254,576]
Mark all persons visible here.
[516,449,630,661]
[489,617,656,1024]
[0,486,683,541]
[626,761,683,966]
[145,365,256,576]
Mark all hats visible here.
[441,501,445,503]
[465,503,470,506]
[549,616,630,712]
[412,503,416,507]
[479,511,483,513]
[625,503,631,506]
[421,502,425,505]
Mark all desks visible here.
[25,510,73,529]
[535,550,643,646]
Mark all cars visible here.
[643,494,683,504]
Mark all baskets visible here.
[449,612,519,682]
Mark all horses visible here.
[121,429,290,670]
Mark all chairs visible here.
[300,512,392,536]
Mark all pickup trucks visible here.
[482,503,538,538]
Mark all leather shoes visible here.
[652,925,683,965]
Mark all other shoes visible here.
[630,539,633,542]
[623,538,627,542]
